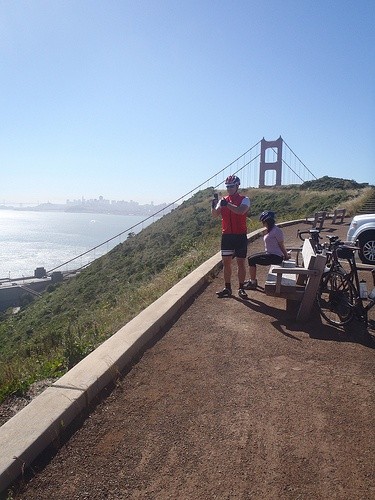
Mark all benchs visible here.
[300,209,346,228]
[264,239,327,322]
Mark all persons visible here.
[243,210,291,289]
[211,175,250,298]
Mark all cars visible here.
[347,214,375,266]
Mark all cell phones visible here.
[213,193,219,203]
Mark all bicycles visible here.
[296,217,375,327]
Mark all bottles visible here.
[368,287,375,301]
[360,279,368,299]
[321,248,326,255]
[287,250,292,256]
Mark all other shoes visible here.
[215,287,232,297]
[237,288,248,298]
[243,278,257,289]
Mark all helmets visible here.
[258,210,274,222]
[225,176,241,186]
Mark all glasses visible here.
[226,185,236,188]
